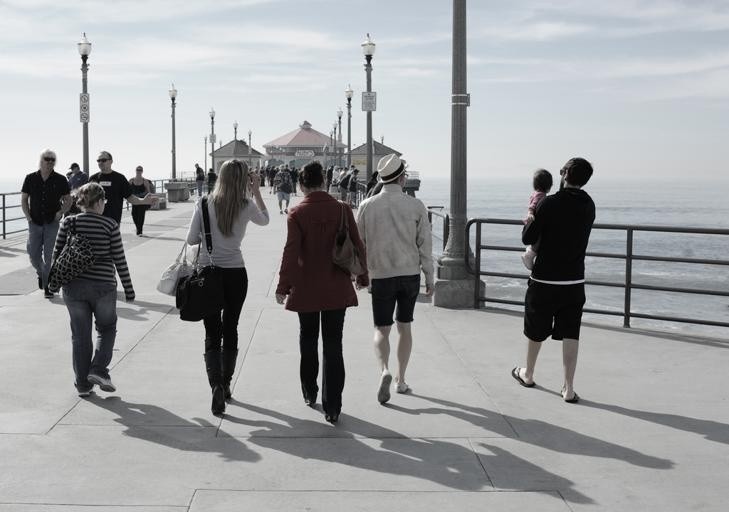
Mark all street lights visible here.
[199,105,256,183]
[165,82,181,187]
[75,30,92,183]
[321,84,354,185]
[357,30,380,189]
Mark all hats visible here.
[374,153,410,183]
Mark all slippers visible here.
[559,388,580,403]
[512,366,533,388]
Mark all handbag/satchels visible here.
[45,214,94,294]
[279,181,293,195]
[330,203,366,274]
[155,259,227,322]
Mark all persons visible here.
[187,159,269,415]
[52,181,135,397]
[520,169,552,270]
[21,149,160,297]
[357,153,434,404]
[275,160,369,422]
[512,157,596,403]
[195,163,378,214]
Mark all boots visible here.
[222,348,238,402]
[204,347,226,416]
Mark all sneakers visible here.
[86,368,118,392]
[76,386,94,399]
[394,380,408,391]
[325,409,341,424]
[377,370,393,406]
[305,396,317,407]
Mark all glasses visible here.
[95,158,108,162]
[43,157,55,161]
